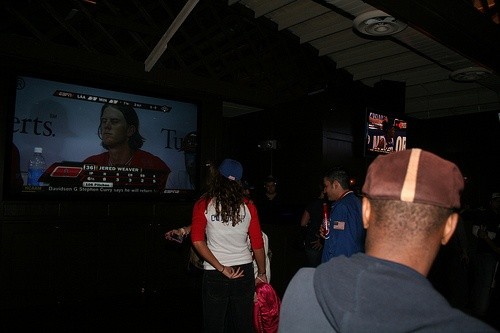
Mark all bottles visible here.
[322,203,330,236]
[27,147,46,186]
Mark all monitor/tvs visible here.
[364,107,410,156]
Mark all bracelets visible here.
[180,226,189,236]
[258,272,266,274]
[221,265,225,273]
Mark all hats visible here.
[218,159,243,181]
[362,149,464,209]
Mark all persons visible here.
[278,148,500,333]
[192,158,268,333]
[70,103,171,191]
[165,170,367,292]
[374,120,396,152]
[436,177,500,327]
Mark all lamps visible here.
[451,67,493,82]
[353,10,408,36]
